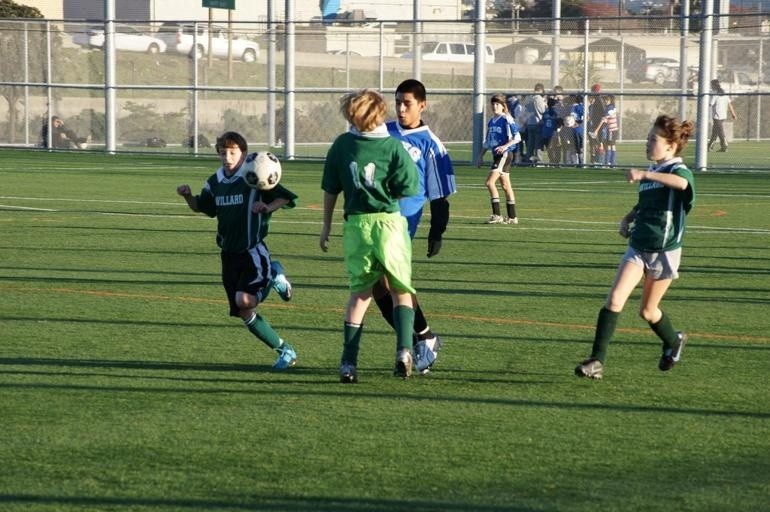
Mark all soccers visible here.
[564,116,575,127]
[242,152,282,190]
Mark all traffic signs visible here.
[202,0,236,9]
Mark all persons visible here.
[317,89,417,383]
[176,130,297,370]
[474,93,520,226]
[508,82,619,171]
[374,78,458,374]
[706,80,738,153]
[42,116,92,151]
[574,114,695,383]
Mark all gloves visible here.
[426,231,443,258]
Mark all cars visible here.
[625,57,689,85]
[718,70,753,85]
[72,23,167,55]
[326,49,362,55]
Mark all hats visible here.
[593,84,601,93]
[562,116,575,128]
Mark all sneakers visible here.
[339,362,359,384]
[502,216,519,225]
[393,348,413,378]
[510,160,617,168]
[271,260,293,302]
[574,357,604,381]
[708,143,729,152]
[484,214,504,224]
[659,330,687,371]
[272,347,298,369]
[412,333,441,376]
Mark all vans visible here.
[399,41,497,64]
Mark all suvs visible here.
[154,17,262,65]
